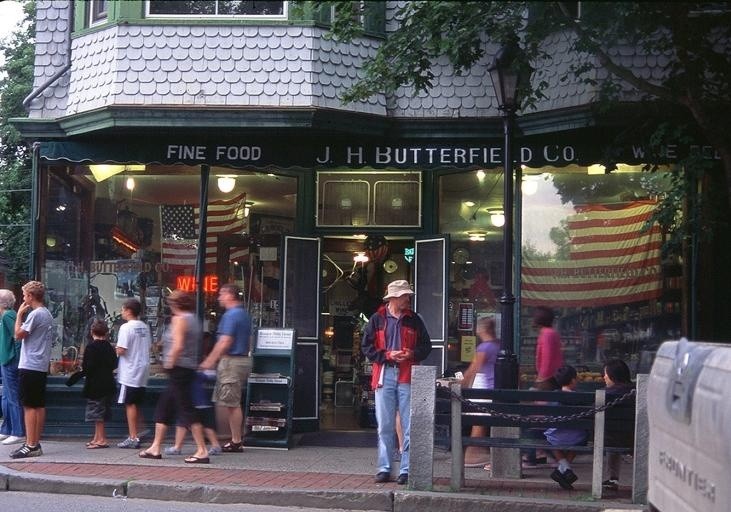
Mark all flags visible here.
[159,193,250,268]
[520,201,665,309]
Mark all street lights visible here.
[486,26,536,480]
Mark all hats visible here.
[383,280,415,300]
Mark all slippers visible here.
[85,440,110,449]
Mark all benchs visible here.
[451,384,635,498]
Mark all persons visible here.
[165,368,222,455]
[83,320,118,449]
[116,299,152,449]
[10,281,53,458]
[533,305,565,463]
[201,284,253,452]
[0,289,27,445]
[362,281,431,486]
[138,290,210,463]
[461,318,501,469]
[592,360,635,488]
[543,366,591,489]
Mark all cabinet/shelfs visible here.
[243,350,296,448]
[520,236,691,366]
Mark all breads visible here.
[519,369,603,384]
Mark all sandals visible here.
[140,439,245,463]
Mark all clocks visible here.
[453,247,471,265]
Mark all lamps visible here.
[353,252,368,262]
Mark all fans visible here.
[320,254,343,294]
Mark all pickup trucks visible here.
[643,336,729,512]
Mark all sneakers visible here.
[0,432,26,445]
[602,479,619,488]
[117,436,142,448]
[522,453,548,469]
[397,474,409,485]
[551,469,577,490]
[375,471,391,482]
[9,443,42,458]
